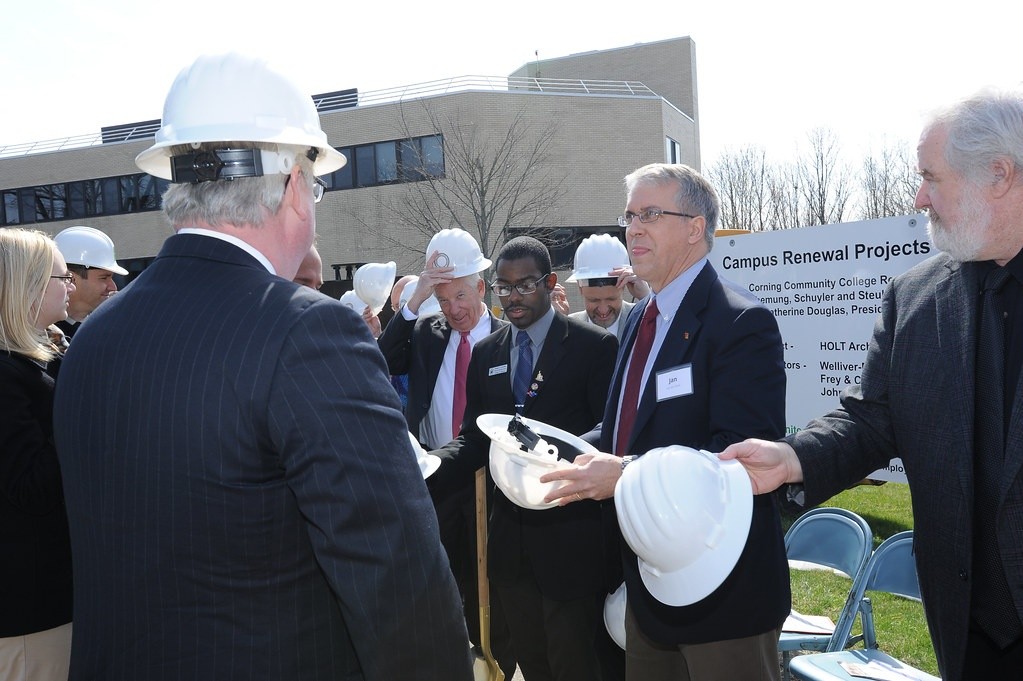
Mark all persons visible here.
[0,225,130,679]
[293,242,322,290]
[363,228,651,681]
[54,48,474,681]
[540,162,791,681]
[718,88,1023,681]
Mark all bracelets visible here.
[621,456,632,473]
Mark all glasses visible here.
[617,207,695,227]
[391,305,399,312]
[46,273,73,284]
[489,274,550,296]
[285,169,327,204]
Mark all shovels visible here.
[469,465,506,681]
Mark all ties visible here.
[391,373,408,416]
[513,330,533,413]
[615,298,659,461]
[970,267,1022,648]
[452,332,471,440]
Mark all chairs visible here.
[788,530,943,681]
[777,502,873,681]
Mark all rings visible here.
[575,493,582,500]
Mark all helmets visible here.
[424,229,492,279]
[135,54,347,182]
[406,432,441,481]
[53,226,129,276]
[476,414,598,510]
[603,578,627,650]
[614,446,753,608]
[353,261,397,318]
[565,233,630,287]
[399,279,442,317]
[340,290,368,317]
